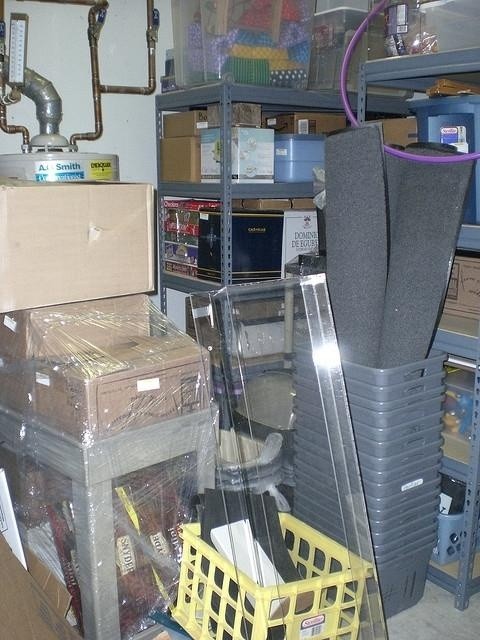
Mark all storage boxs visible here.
[161,108,421,188]
[1,181,152,326]
[157,196,321,284]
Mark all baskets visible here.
[169,509,374,640]
[430,503,480,568]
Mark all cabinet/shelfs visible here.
[335,47,478,612]
[151,80,406,618]
